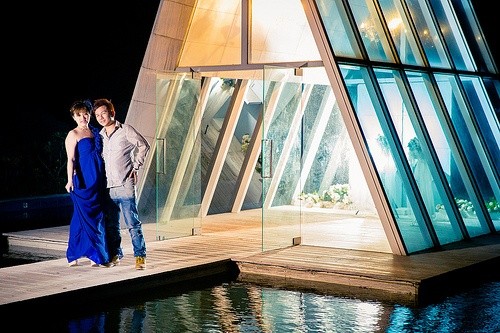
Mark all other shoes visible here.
[69,259,78,266]
[91,260,99,267]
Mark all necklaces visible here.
[79,127,89,133]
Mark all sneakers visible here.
[111,254,120,266]
[135,256,146,270]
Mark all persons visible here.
[93,98,151,270]
[64,100,109,268]
